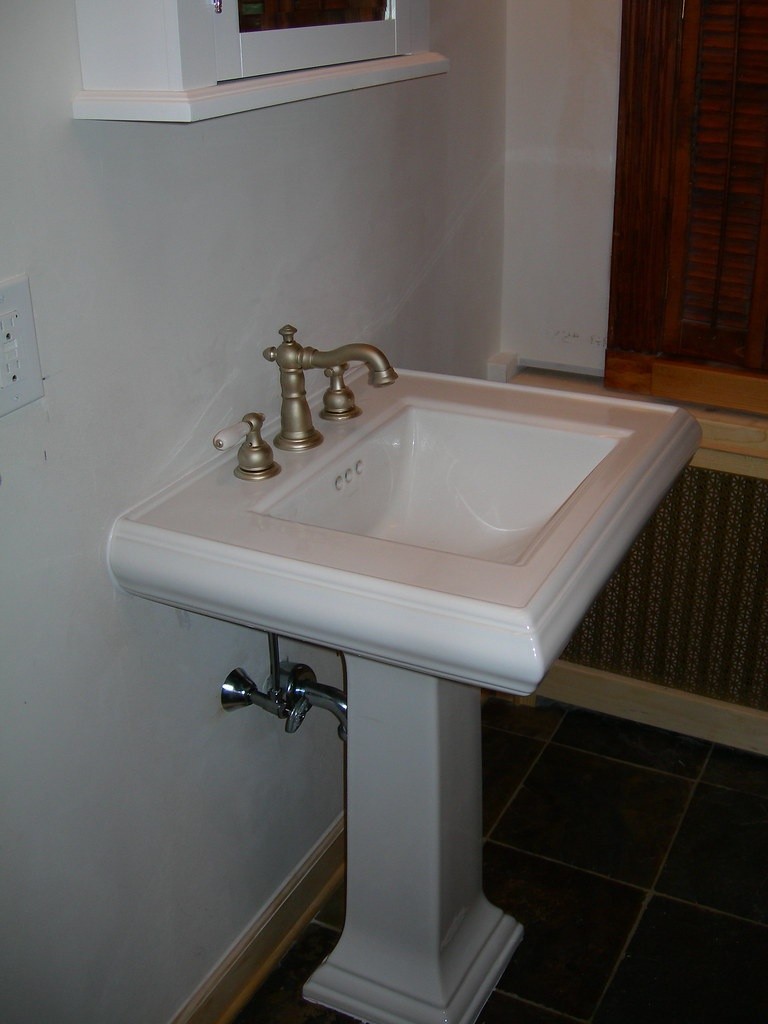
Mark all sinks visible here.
[244,393,706,697]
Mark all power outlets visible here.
[0,271,45,419]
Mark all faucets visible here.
[261,324,399,452]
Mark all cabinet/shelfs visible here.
[76,0,429,92]
[603,0,768,421]
